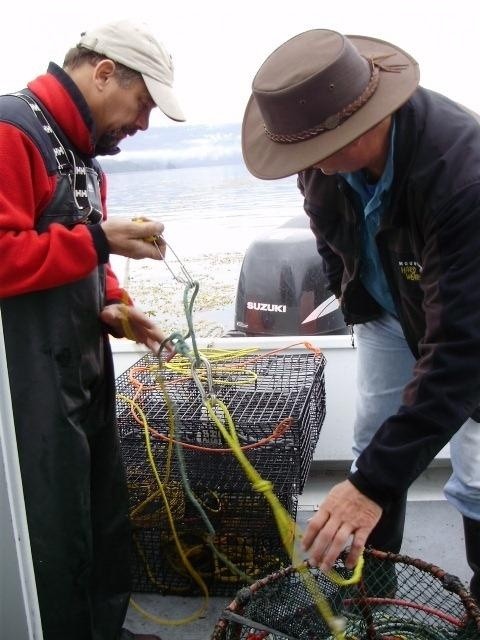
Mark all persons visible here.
[243,27,478,636]
[1,22,178,639]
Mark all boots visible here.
[454,516,480,639]
[340,488,407,597]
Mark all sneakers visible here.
[120,627,161,640]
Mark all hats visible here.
[78,22,187,122]
[241,29,419,180]
